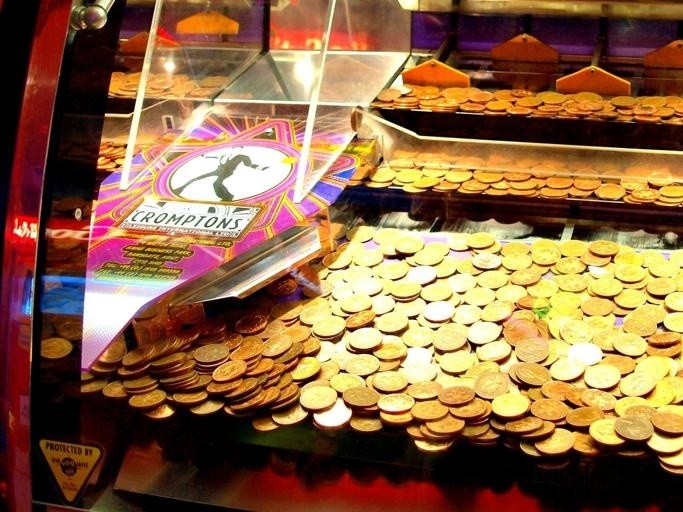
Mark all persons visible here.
[173,145,268,202]
